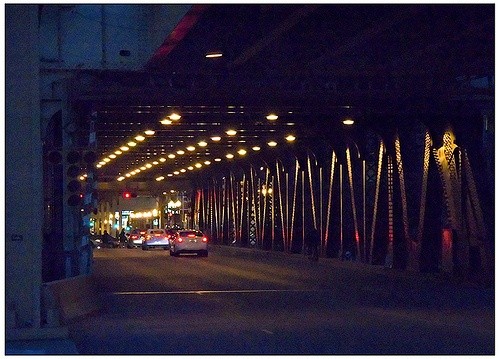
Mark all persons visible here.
[305,225,321,260]
[120,228,125,248]
[103,230,119,248]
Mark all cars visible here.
[142,229,169,250]
[168,229,208,257]
[127,230,147,249]
[90,235,120,247]
